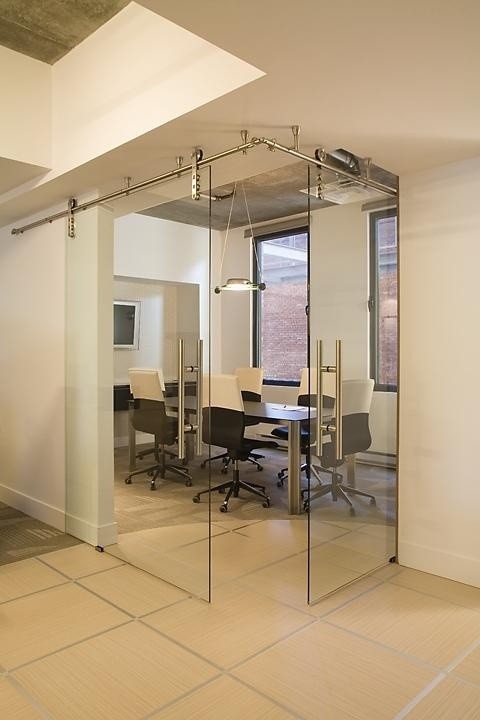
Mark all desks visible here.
[125,395,336,516]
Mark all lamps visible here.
[216,177,266,296]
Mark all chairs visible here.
[298,378,378,515]
[271,367,344,489]
[124,369,193,490]
[127,365,180,460]
[221,366,264,462]
[194,371,274,511]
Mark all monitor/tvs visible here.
[113,300,141,351]
